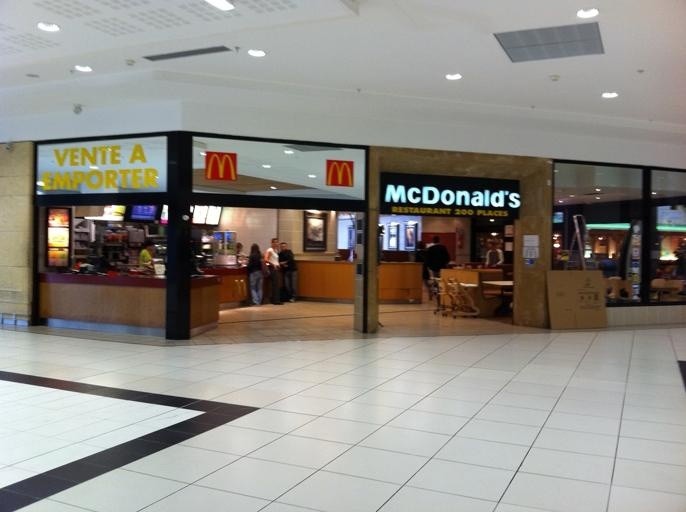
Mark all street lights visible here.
[263,266,271,278]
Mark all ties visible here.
[123,204,163,223]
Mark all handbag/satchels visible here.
[268,261,272,265]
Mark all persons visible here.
[264,238,283,305]
[484,241,505,267]
[236,242,244,264]
[137,241,155,269]
[279,243,298,303]
[246,243,264,305]
[426,235,451,294]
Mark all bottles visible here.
[144,241,156,245]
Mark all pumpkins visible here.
[564,234,584,270]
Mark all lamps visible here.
[428,268,686,320]
